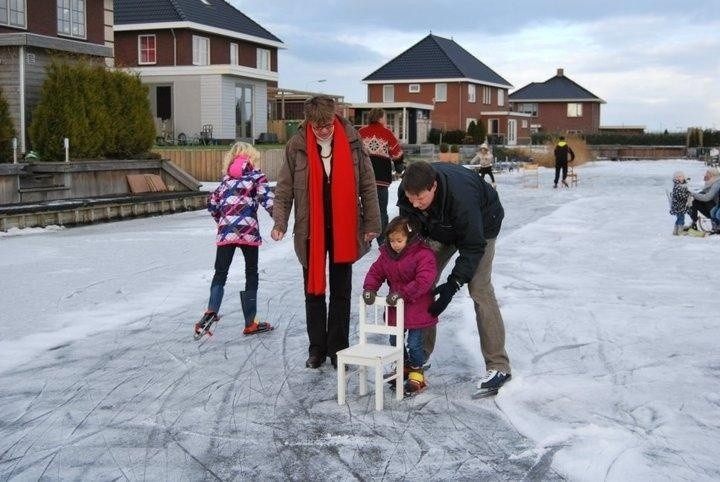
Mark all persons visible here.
[553,136,575,189]
[272,95,382,371]
[710,195,720,235]
[194,141,275,341]
[471,143,497,189]
[710,147,719,168]
[669,170,691,236]
[358,107,405,246]
[363,216,441,396]
[395,161,511,390]
[683,169,720,232]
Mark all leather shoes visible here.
[306,354,325,368]
[331,356,347,370]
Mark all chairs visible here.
[523,164,544,188]
[335,298,404,411]
[565,162,578,188]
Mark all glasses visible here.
[313,123,334,130]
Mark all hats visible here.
[480,142,488,150]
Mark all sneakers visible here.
[477,370,511,387]
[243,322,271,333]
[389,368,408,382]
[196,312,216,335]
[562,180,568,187]
[406,374,424,390]
[553,184,557,187]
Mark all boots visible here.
[673,225,678,235]
[677,225,686,234]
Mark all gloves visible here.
[386,293,401,304]
[427,278,459,318]
[363,291,376,304]
